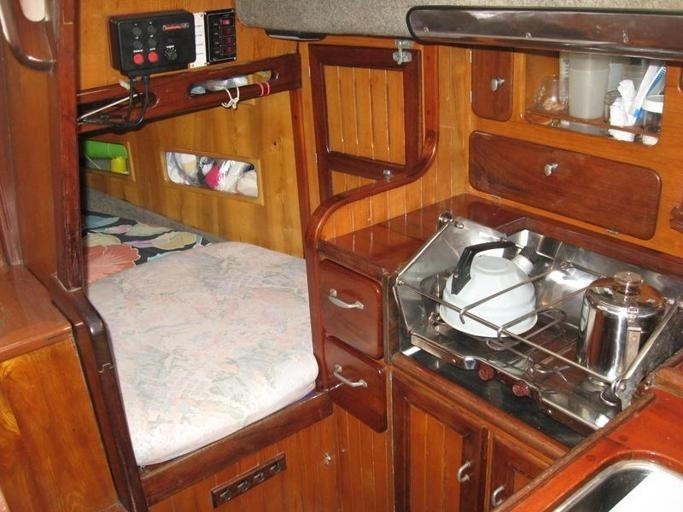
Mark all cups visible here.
[535,74,569,116]
[602,91,643,143]
[567,52,610,122]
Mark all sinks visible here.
[550,458,683,511]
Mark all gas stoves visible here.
[387,212,683,438]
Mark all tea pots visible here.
[438,240,540,339]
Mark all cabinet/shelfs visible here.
[389,364,572,512]
[314,249,410,512]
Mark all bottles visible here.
[642,94,664,145]
